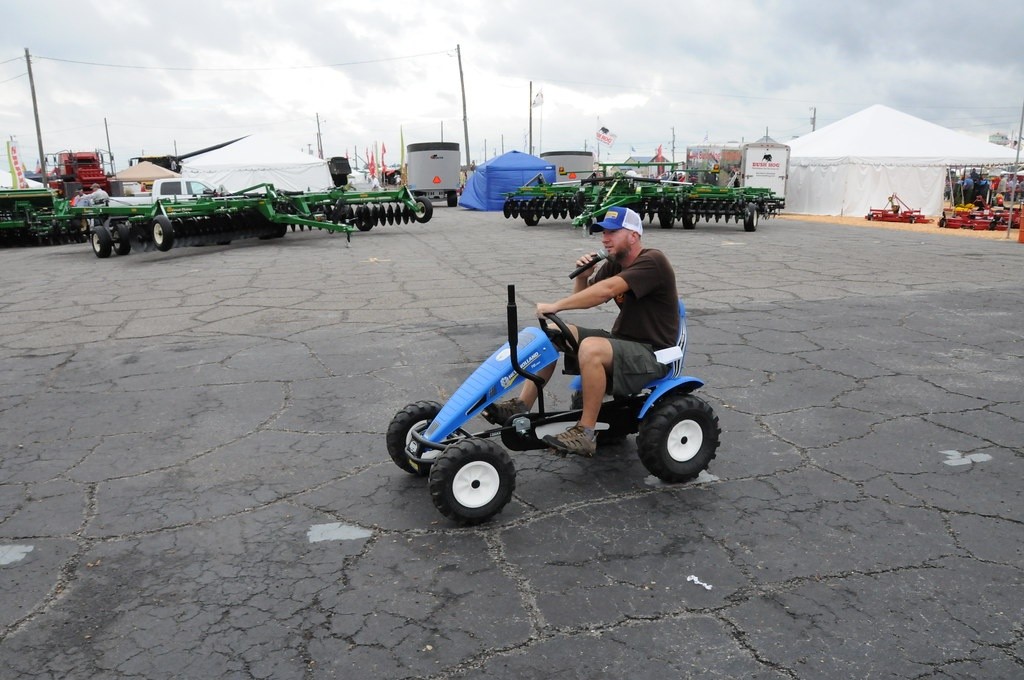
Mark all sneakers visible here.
[486,398,527,425]
[543,421,596,457]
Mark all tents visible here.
[110,160,183,196]
[459,149,556,211]
[179,132,338,193]
[768,103,1023,219]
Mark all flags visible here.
[595,118,617,148]
[532,88,544,108]
[368,152,375,176]
[7,141,25,190]
[381,142,388,173]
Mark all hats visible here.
[589,206,643,237]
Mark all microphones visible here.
[567,248,611,280]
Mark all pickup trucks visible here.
[107,178,220,207]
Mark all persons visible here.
[86,182,109,203]
[480,204,683,456]
[74,185,93,207]
[170,159,177,171]
[946,168,1024,207]
[141,183,146,192]
[372,174,379,191]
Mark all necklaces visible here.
[0,169,44,189]
